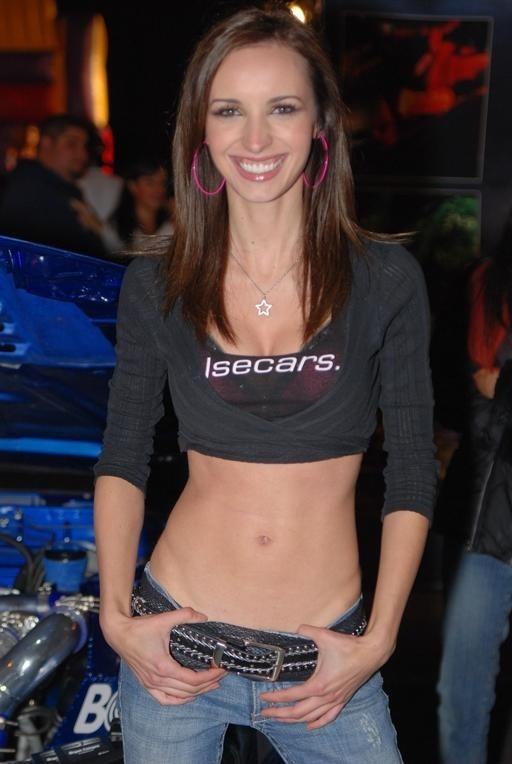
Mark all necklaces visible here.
[204,229,314,314]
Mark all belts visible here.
[130,590,369,683]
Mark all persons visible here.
[91,8,436,764]
[465,247,511,376]
[435,330,511,762]
[97,155,183,259]
[0,111,109,258]
